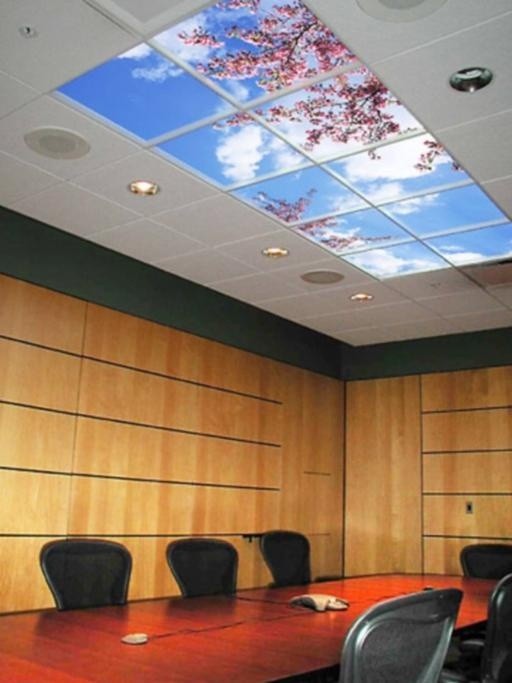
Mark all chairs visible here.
[335,585,460,683]
[260,529,344,588]
[460,543,512,581]
[39,538,132,610]
[438,573,512,683]
[165,538,239,596]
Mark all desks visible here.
[0,569,493,683]
